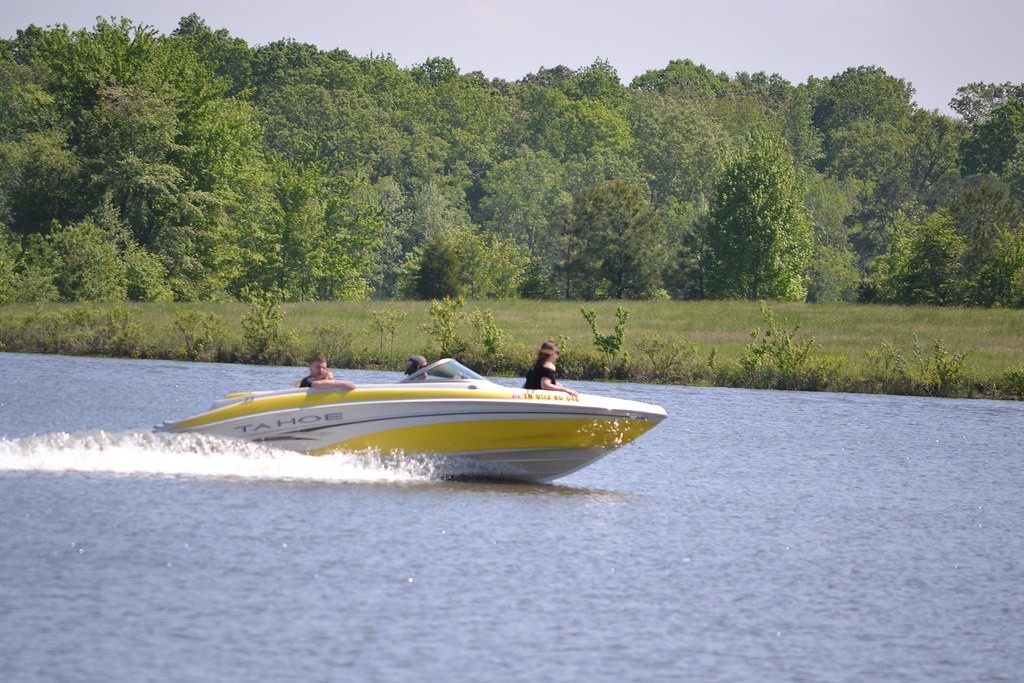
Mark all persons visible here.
[522,342,579,397]
[404,356,428,380]
[300,356,356,389]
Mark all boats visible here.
[149,357,669,488]
